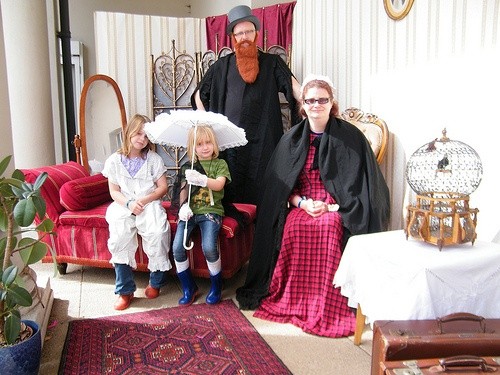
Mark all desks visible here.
[332,229,500,346]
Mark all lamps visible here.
[405,128,483,252]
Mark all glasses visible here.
[304,97,328,105]
[233,29,257,37]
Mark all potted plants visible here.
[0,154,54,375]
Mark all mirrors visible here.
[74,75,128,176]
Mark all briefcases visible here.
[372,312,500,375]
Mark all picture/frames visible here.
[383,0,415,21]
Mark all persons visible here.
[235,79,391,338]
[172,125,232,306]
[190,5,303,204]
[101,113,172,310]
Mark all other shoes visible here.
[115,293,133,310]
[144,284,160,298]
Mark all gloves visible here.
[186,170,207,187]
[178,202,193,222]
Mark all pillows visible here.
[59,173,110,211]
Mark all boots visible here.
[176,266,200,307]
[205,270,223,305]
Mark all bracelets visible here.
[127,200,134,206]
[298,199,303,208]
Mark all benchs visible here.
[15,161,256,285]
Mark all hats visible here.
[225,5,260,36]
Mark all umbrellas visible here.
[143,109,248,251]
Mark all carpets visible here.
[57,298,293,375]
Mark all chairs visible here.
[335,107,389,166]
